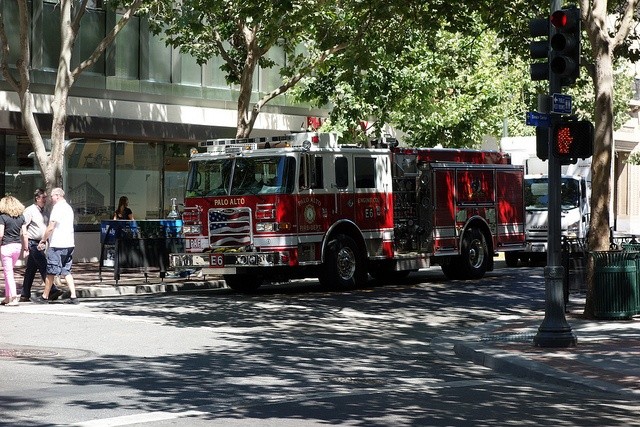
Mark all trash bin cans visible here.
[589,249,639,319]
[622,243,640,315]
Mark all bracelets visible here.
[23,247,29,251]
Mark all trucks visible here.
[505,175,591,267]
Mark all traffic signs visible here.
[553,93,572,114]
[556,115,578,165]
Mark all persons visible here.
[0,195,29,306]
[20,188,64,301]
[28,187,79,304]
[111,195,136,220]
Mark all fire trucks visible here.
[168,131,525,291]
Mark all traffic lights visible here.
[550,5,580,84]
[530,16,549,80]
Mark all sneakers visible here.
[29,296,48,304]
[5,299,19,306]
[63,298,80,305]
[0,296,8,304]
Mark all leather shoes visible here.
[50,288,63,300]
[19,297,31,302]
